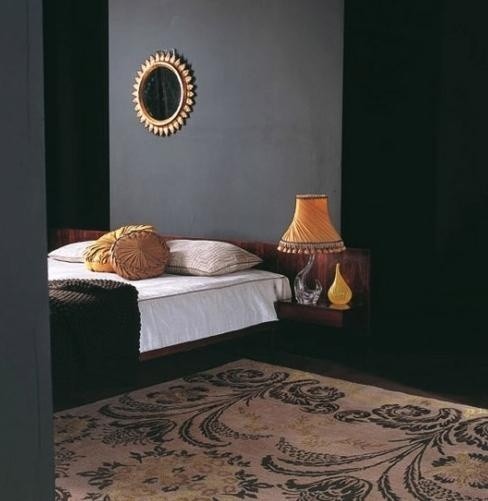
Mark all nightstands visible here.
[274,294,367,365]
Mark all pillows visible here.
[162,239,263,277]
[85,223,154,273]
[109,232,170,279]
[48,240,95,263]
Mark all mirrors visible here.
[132,50,194,137]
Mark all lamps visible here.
[277,194,347,306]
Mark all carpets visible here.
[54,358,486,500]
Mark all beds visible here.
[46,225,370,411]
